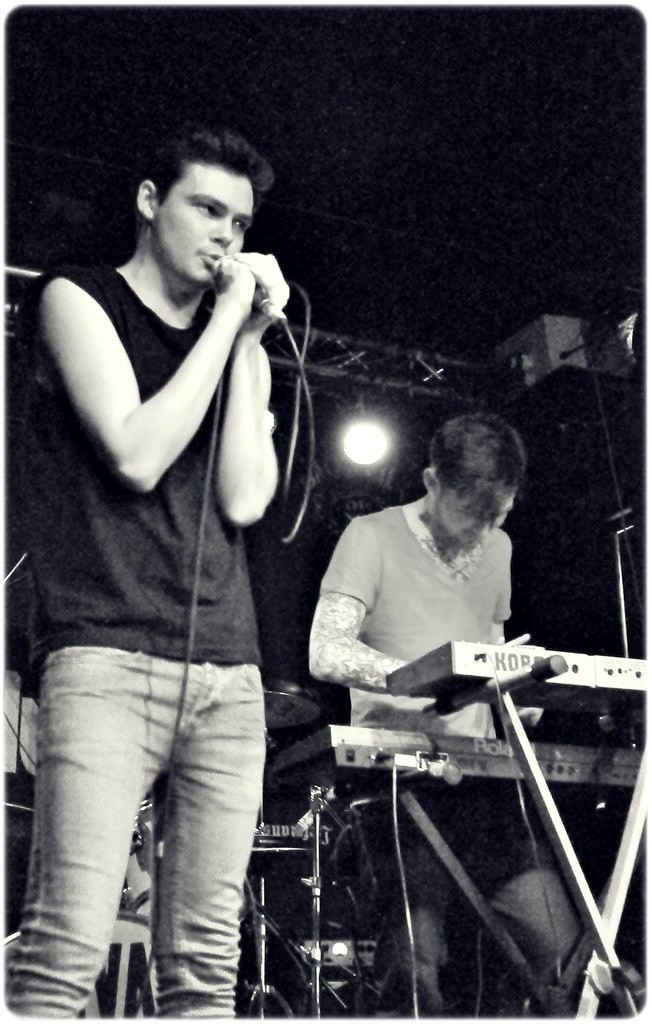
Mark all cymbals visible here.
[263,689,323,732]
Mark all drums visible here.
[1,901,170,1021]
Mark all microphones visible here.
[252,287,286,326]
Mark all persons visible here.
[309,418,585,1018]
[5,128,289,1019]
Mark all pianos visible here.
[267,720,646,822]
[379,633,645,714]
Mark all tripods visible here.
[247,783,348,1018]
